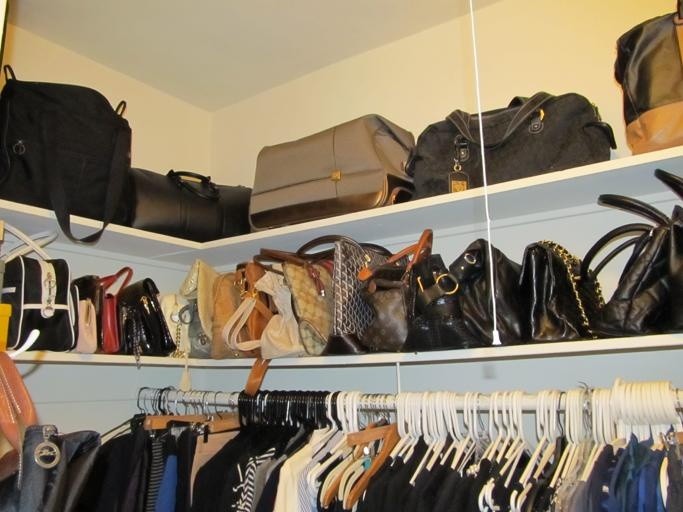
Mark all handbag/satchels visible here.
[615,11,683,155]
[2,168,683,358]
[406,92,616,199]
[0,77,135,223]
[130,168,252,239]
[246,110,418,232]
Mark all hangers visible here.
[97,377,683,512]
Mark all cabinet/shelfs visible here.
[0,144,682,369]
